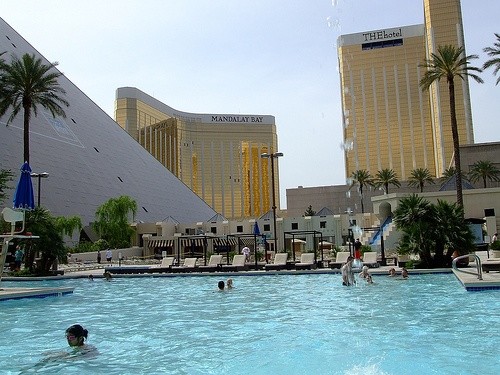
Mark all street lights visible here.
[29,171,49,208]
[259,152,284,254]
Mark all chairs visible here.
[147,255,176,273]
[264,252,289,272]
[169,257,198,273]
[329,251,350,269]
[294,252,317,271]
[198,254,224,273]
[222,254,248,272]
[352,252,380,269]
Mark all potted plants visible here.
[486,239,500,258]
[396,236,409,262]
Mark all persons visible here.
[14,246,24,270]
[366,275,373,285]
[401,268,408,277]
[491,232,499,249]
[89,275,93,280]
[217,281,225,291]
[359,266,368,278]
[67,324,87,346]
[226,278,234,288]
[354,238,362,260]
[103,271,112,280]
[241,245,250,262]
[97,251,101,263]
[343,256,357,286]
[388,268,396,276]
[24,239,36,273]
[106,248,113,266]
[76,258,81,264]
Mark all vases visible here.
[469,252,476,261]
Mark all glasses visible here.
[67,335,77,340]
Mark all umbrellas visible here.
[253,221,260,235]
[13,160,34,232]
